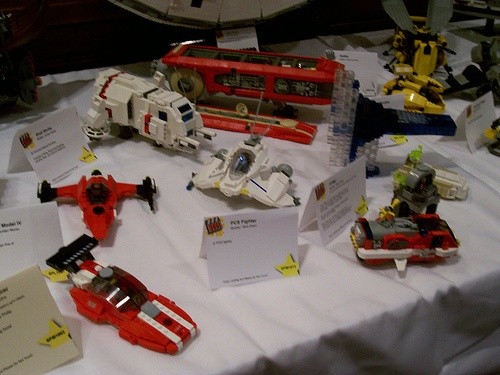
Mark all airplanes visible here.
[186,116,300,208]
[37,168,157,245]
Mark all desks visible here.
[1,25,500,374]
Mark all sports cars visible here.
[46,233,201,355]
